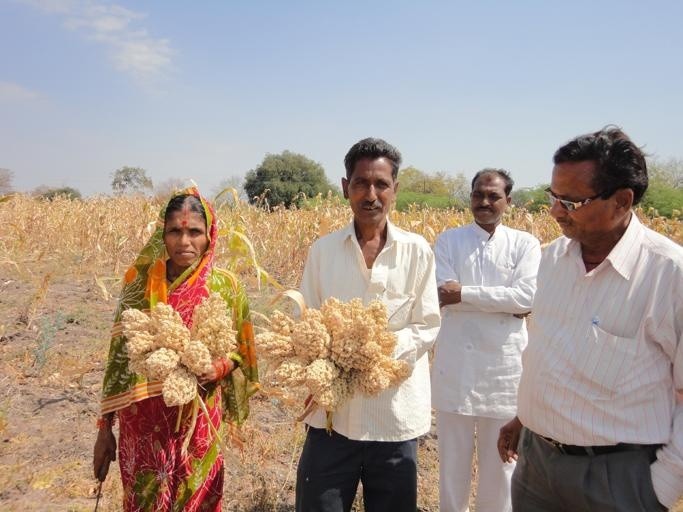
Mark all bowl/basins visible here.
[164,258,174,284]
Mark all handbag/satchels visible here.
[95,417,117,430]
[212,359,230,381]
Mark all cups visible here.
[544,187,612,211]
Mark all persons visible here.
[433,167,543,511]
[496,121,683,511]
[92,184,262,512]
[287,135,443,511]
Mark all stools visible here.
[537,433,659,457]
